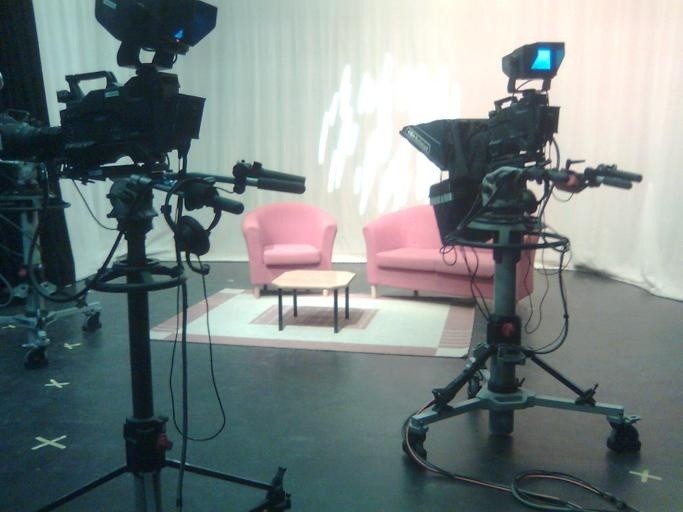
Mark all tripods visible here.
[0,265,292,507]
[404,241,642,452]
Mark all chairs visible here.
[244,203,337,296]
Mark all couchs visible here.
[365,204,542,300]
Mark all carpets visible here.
[136,286,476,359]
